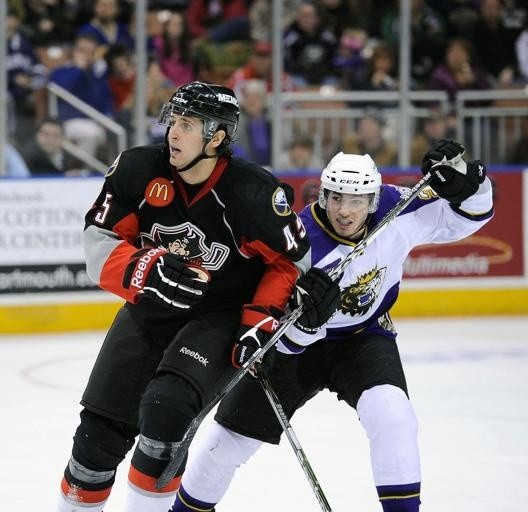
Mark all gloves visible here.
[421,139,488,210]
[120,246,211,316]
[231,304,287,380]
[290,267,343,336]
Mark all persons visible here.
[56,78,312,512]
[170,138,496,512]
[0,0,528,176]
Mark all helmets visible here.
[156,80,240,146]
[317,152,384,215]
